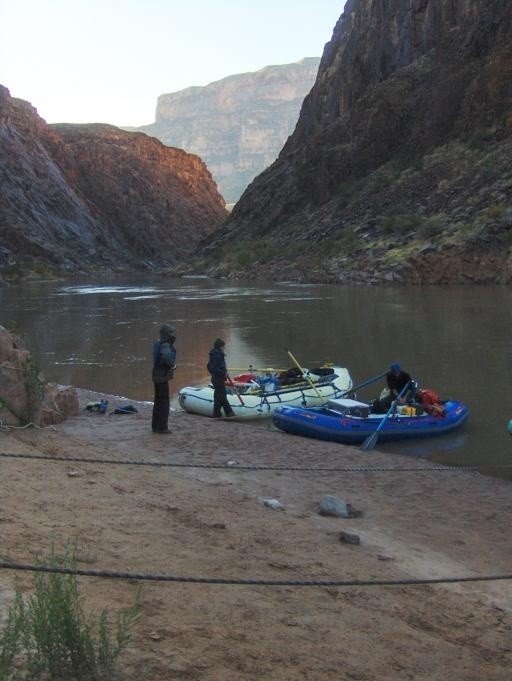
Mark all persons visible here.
[151,324,174,434]
[208,338,235,419]
[379,362,415,414]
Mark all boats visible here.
[178,364,353,422]
[271,396,469,443]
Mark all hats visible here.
[214,338,226,349]
[390,362,401,373]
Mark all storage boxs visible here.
[328,398,369,418]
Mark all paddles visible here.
[360,381,409,449]
